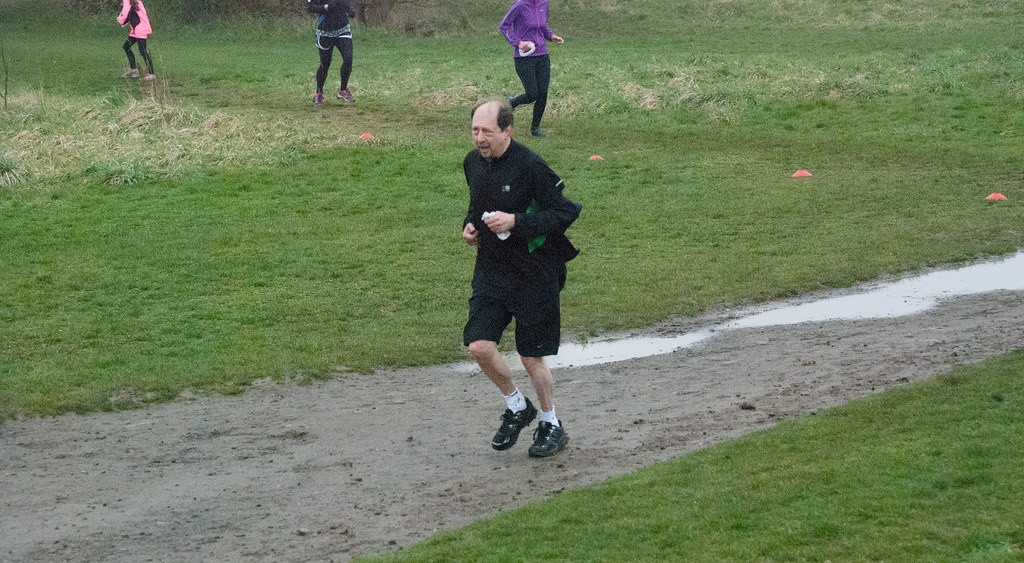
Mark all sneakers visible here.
[138,74,157,80]
[490,395,539,452]
[530,128,547,137]
[121,68,140,77]
[506,97,517,113]
[527,420,570,458]
[313,93,325,108]
[337,89,356,103]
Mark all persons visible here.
[114,0,156,82]
[305,0,357,106]
[501,1,565,139]
[459,98,583,457]
